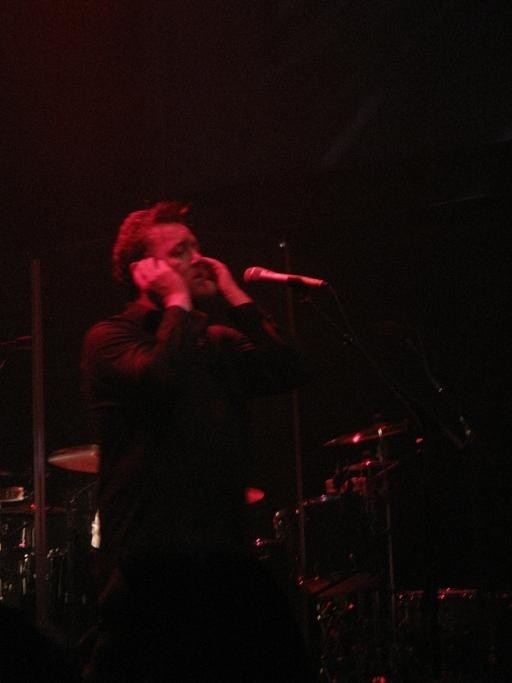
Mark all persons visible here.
[79,202,328,682]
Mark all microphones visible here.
[241,265,328,291]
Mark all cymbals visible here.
[0,487,31,501]
[47,443,99,474]
[336,459,396,470]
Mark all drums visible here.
[254,538,275,562]
[274,495,376,593]
[1,506,65,576]
[325,423,405,447]
